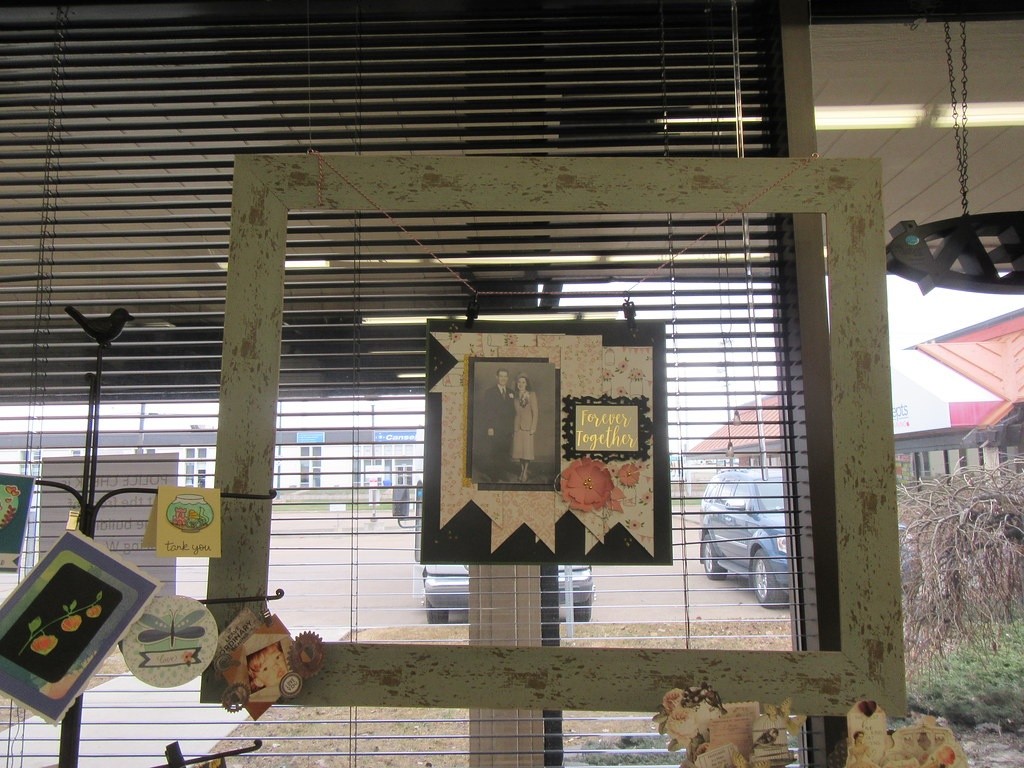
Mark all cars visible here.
[391,475,596,622]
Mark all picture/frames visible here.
[421,318,673,567]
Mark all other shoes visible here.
[519,472,527,482]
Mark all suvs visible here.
[697,465,791,608]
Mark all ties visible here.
[502,388,506,399]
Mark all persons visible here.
[485,368,539,483]
[247,642,289,702]
